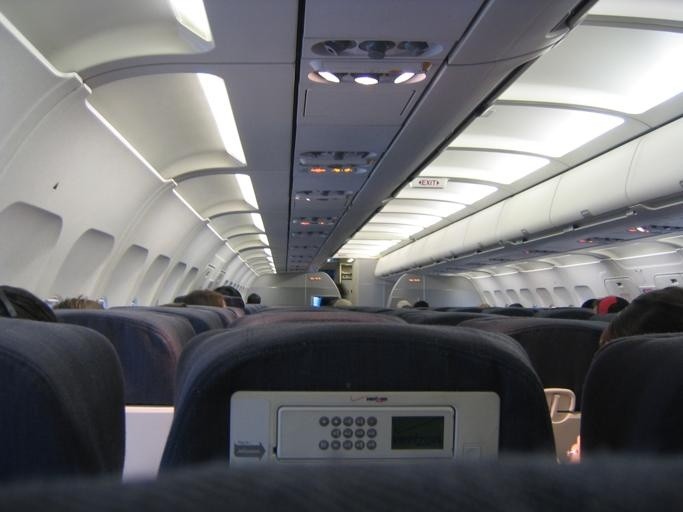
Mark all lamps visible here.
[318,72,415,85]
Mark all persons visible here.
[334,298,353,308]
[479,303,489,308]
[581,285,683,348]
[396,299,411,308]
[160,285,261,308]
[412,300,429,308]
[509,302,523,308]
[0,285,56,322]
[52,297,104,310]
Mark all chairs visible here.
[1,304,682,481]
